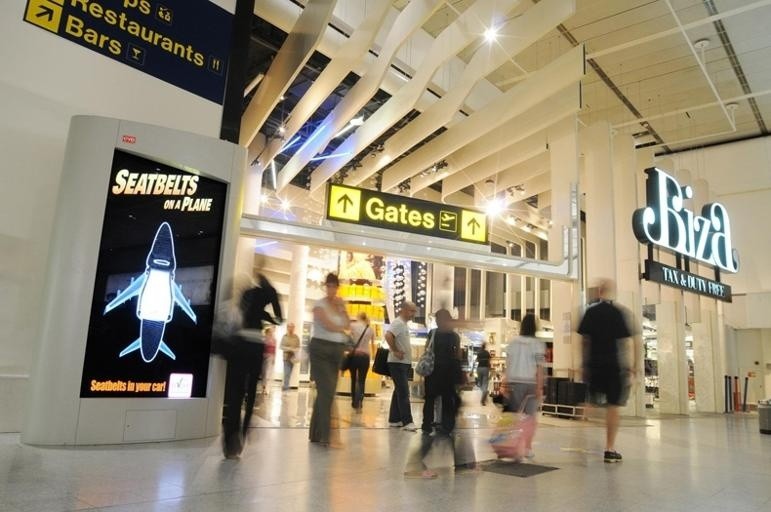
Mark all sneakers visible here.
[422,422,449,432]
[388,421,402,427]
[604,452,621,463]
[403,423,417,431]
[524,449,535,458]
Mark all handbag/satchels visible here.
[371,348,391,377]
[211,272,244,338]
[339,351,354,371]
[415,329,436,377]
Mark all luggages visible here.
[488,395,542,463]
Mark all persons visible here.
[261,326,278,398]
[577,277,637,467]
[212,248,286,460]
[386,300,420,431]
[308,271,350,449]
[504,312,547,459]
[468,342,492,405]
[405,307,486,479]
[348,310,377,415]
[279,321,301,392]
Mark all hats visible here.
[598,282,616,300]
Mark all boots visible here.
[481,391,489,405]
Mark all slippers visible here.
[454,463,486,476]
[404,469,439,479]
